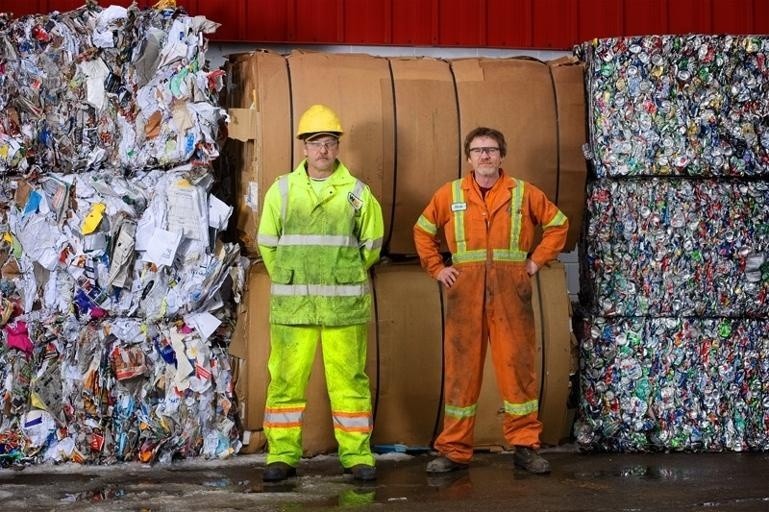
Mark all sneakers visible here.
[343,464,377,482]
[513,447,551,474]
[263,462,296,482]
[425,456,468,472]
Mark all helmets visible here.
[296,104,344,141]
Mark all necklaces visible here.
[309,168,336,183]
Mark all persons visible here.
[254,104,386,482]
[415,126,571,475]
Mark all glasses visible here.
[471,147,499,156]
